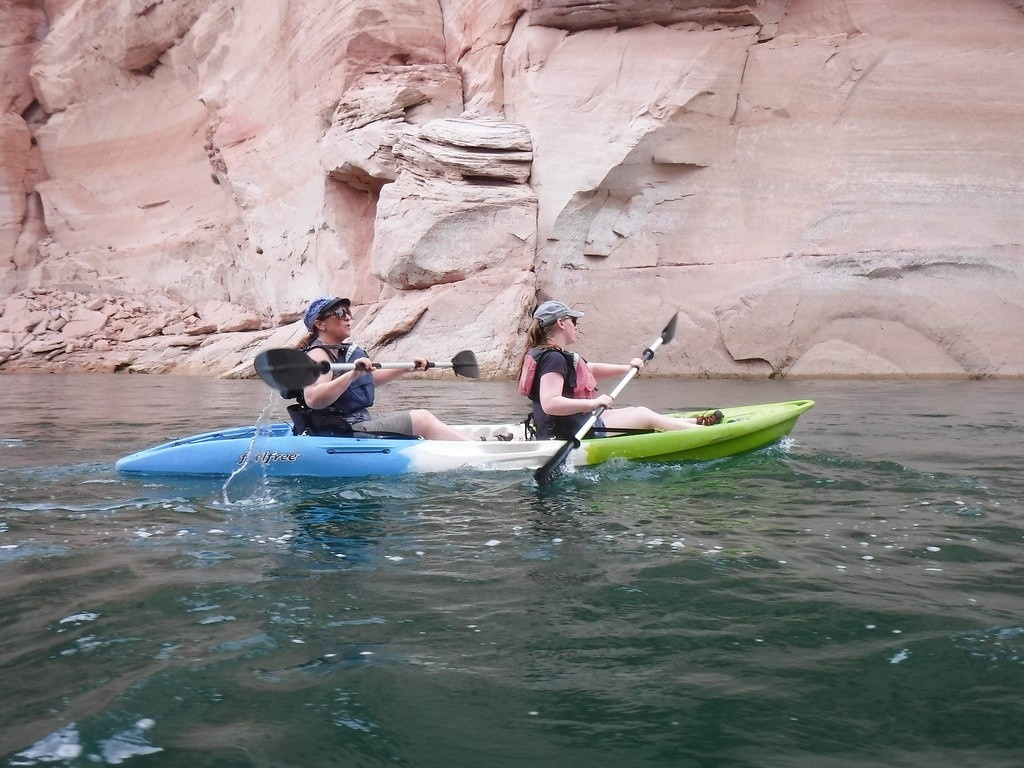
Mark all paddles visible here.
[254,347,479,393]
[533,309,678,480]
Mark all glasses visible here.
[316,306,352,320]
[556,317,578,326]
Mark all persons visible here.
[295,295,514,445]
[530,300,724,440]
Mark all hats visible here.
[533,301,585,327]
[304,296,350,332]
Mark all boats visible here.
[113,399,818,476]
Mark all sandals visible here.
[697,410,722,426]
[480,433,514,442]
